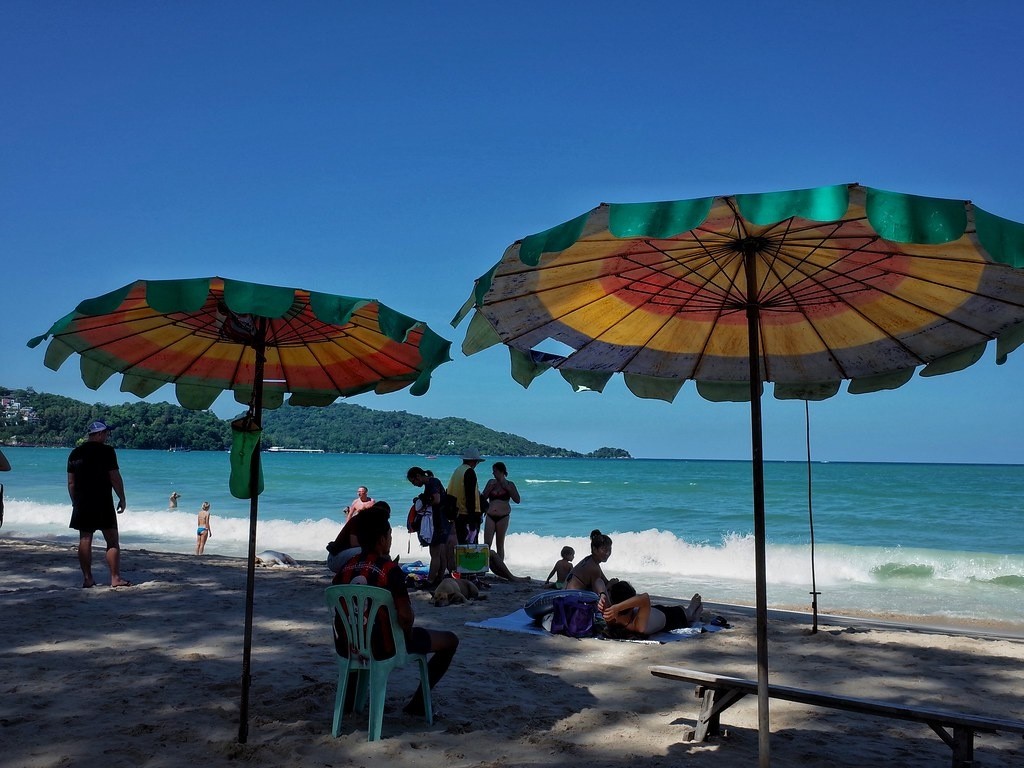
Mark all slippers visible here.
[112,580,131,587]
[711,616,730,628]
[83,580,96,588]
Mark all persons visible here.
[196,502,212,554]
[342,506,350,514]
[561,529,619,595]
[542,546,575,589]
[0,449,13,527]
[346,485,375,523]
[447,446,491,590]
[595,577,703,635]
[407,466,449,590]
[331,515,459,716]
[169,491,181,509]
[66,420,133,587]
[481,462,520,561]
[446,494,531,584]
[327,501,390,573]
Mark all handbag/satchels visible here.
[552,596,596,637]
[407,503,421,533]
[432,478,457,513]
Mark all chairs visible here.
[325,584,433,742]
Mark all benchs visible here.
[648,665,1024,768]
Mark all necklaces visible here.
[624,613,635,626]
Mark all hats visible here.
[88,422,110,434]
[460,448,486,461]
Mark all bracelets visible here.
[598,592,606,597]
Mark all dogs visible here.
[255,550,300,568]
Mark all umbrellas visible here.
[25,275,454,741]
[449,184,1024,768]
